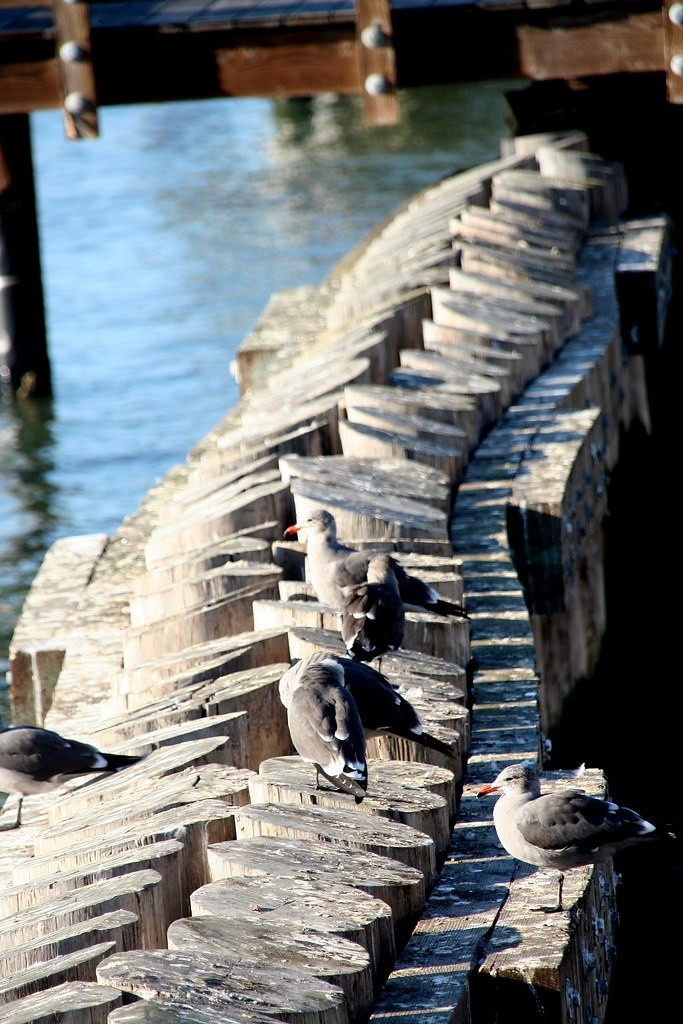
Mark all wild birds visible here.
[277,651,456,761]
[283,509,473,642]
[475,763,656,914]
[339,554,405,673]
[0,725,143,832]
[287,659,368,805]
